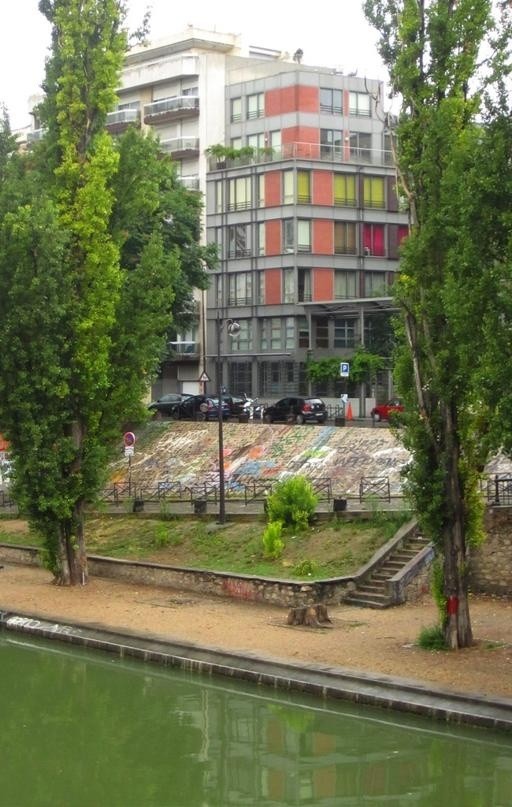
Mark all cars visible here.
[147,394,241,421]
[371,397,407,422]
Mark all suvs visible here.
[263,396,328,424]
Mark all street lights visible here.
[215,308,242,526]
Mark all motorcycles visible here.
[241,392,261,419]
